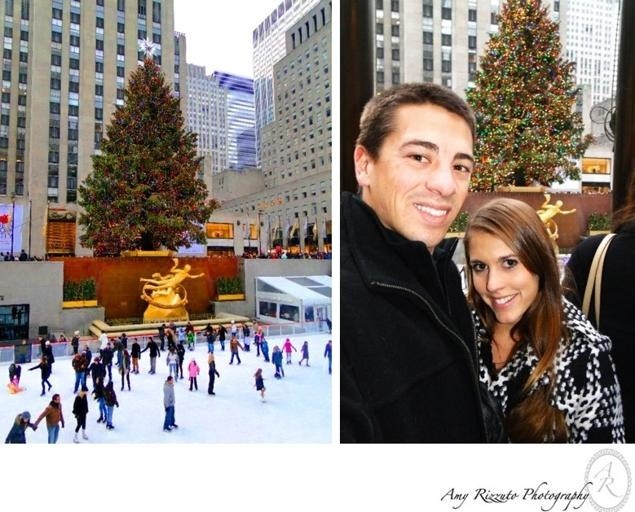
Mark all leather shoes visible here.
[97,416,115,431]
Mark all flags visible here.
[242,210,328,243]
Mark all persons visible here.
[458,194,627,441]
[341,79,510,444]
[0,319,332,444]
[535,190,579,225]
[559,158,635,441]
[264,306,331,319]
[239,251,331,260]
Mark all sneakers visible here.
[74,429,89,443]
[163,424,178,433]
